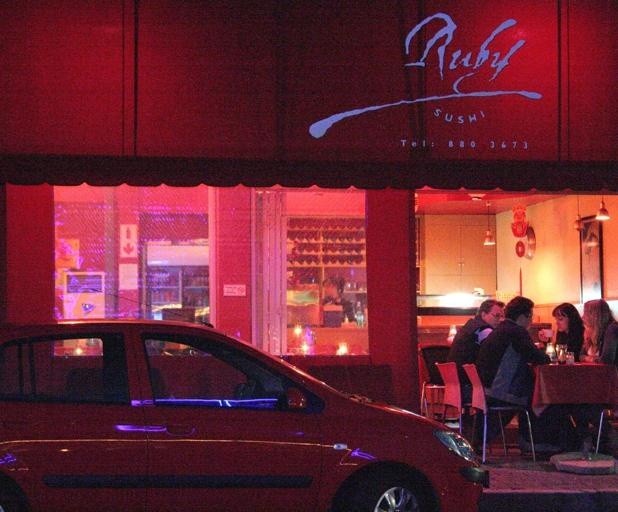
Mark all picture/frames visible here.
[578,215,604,305]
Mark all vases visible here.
[544,343,557,362]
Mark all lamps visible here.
[483,200,496,246]
[594,187,610,220]
[573,194,584,232]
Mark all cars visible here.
[0,314,491,512]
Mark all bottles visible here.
[547,343,575,365]
[288,219,365,293]
[184,272,209,308]
[151,272,178,305]
[448,324,456,345]
[355,301,364,329]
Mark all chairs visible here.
[435,361,493,455]
[462,364,536,465]
[416,344,462,422]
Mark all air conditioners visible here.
[62,272,105,320]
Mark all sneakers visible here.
[522,439,561,456]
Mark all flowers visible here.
[538,329,554,343]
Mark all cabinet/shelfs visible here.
[286,216,368,295]
[418,215,497,309]
[141,239,210,358]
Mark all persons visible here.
[446,296,617,461]
[322,274,356,327]
